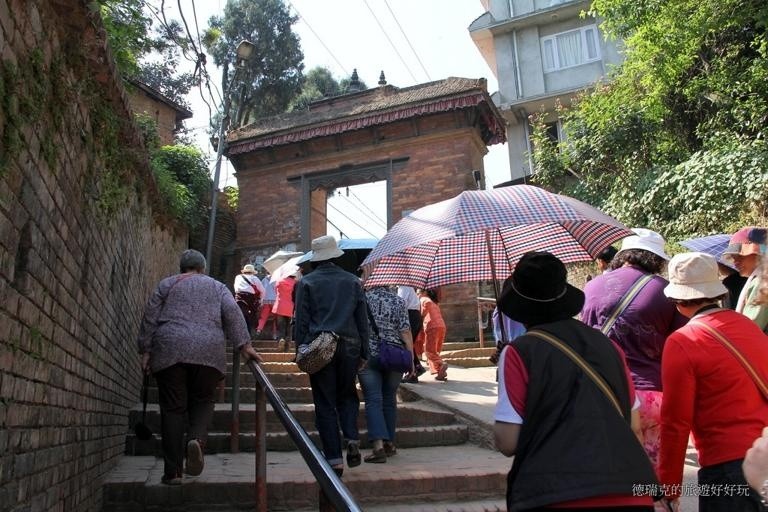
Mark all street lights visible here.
[202,40,257,282]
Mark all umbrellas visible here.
[354,186,639,344]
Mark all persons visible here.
[136,248,264,487]
[231,234,448,478]
[491,227,768,511]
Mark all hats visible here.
[240,264,258,275]
[613,227,670,263]
[307,236,346,263]
[724,226,767,262]
[662,252,729,302]
[496,249,586,327]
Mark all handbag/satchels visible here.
[294,330,340,376]
[373,339,413,374]
[251,283,263,300]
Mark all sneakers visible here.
[160,472,183,485]
[185,438,205,477]
[278,339,286,353]
[435,362,449,382]
[401,375,419,383]
[330,461,344,479]
[345,439,363,468]
[363,447,387,464]
[387,440,401,457]
[416,363,427,376]
